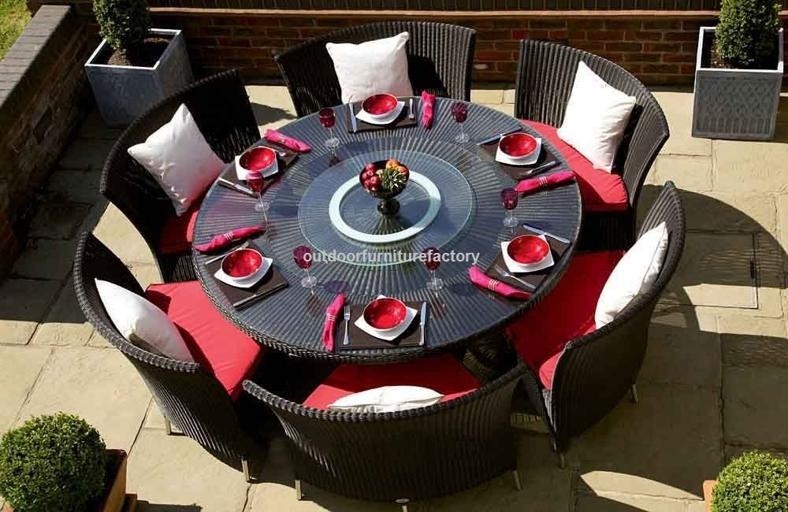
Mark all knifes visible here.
[418,302,427,346]
[476,126,523,146]
[349,103,357,133]
[523,225,571,244]
[218,177,258,198]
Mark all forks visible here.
[519,161,556,177]
[343,305,350,345]
[408,98,416,119]
[259,145,290,157]
[493,264,536,290]
[205,242,250,266]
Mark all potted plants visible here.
[0,412,137,511]
[701,446,787,511]
[689,0,784,140]
[81,1,199,126]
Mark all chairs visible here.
[273,20,476,122]
[242,355,533,512]
[494,181,688,470]
[510,36,673,259]
[71,230,315,483]
[96,68,262,286]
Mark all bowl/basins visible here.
[363,298,407,332]
[222,248,262,280]
[361,93,398,118]
[238,146,275,173]
[498,132,538,159]
[506,235,550,265]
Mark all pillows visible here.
[327,386,440,412]
[94,278,193,364]
[560,62,636,175]
[128,105,228,219]
[594,222,669,329]
[323,32,413,105]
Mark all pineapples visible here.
[376,168,407,193]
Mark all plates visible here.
[494,137,542,165]
[234,154,279,181]
[354,295,417,342]
[355,101,406,125]
[500,234,555,273]
[214,257,273,288]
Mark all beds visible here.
[192,95,584,393]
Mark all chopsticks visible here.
[232,282,294,312]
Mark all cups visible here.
[318,107,340,146]
[499,185,519,227]
[450,100,470,144]
[293,246,317,287]
[423,247,444,291]
[246,171,271,211]
